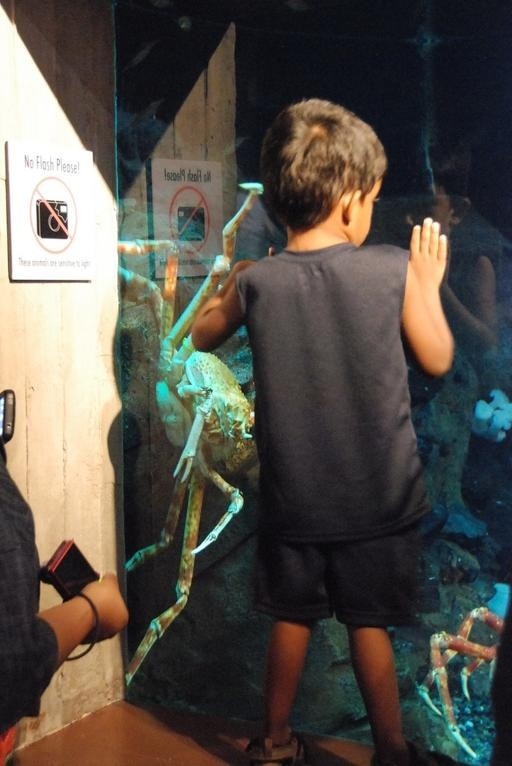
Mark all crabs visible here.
[125,180,265,690]
[414,606,506,758]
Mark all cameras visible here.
[45,539,100,602]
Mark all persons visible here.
[387,150,500,364]
[189,98,454,765]
[1,454,133,732]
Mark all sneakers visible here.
[369,739,454,766]
[245,729,307,766]
